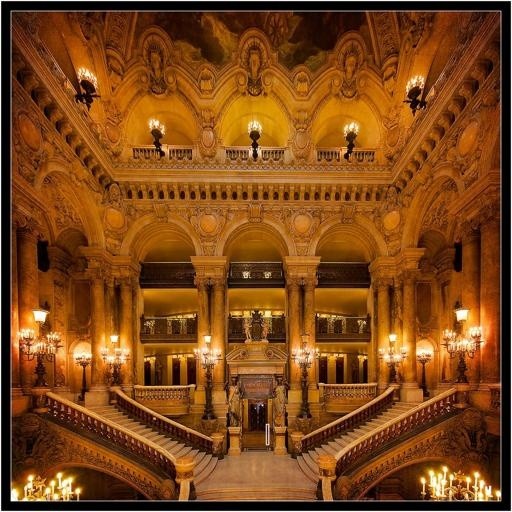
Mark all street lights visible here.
[378,334,409,385]
[11,471,82,501]
[74,349,93,401]
[441,307,483,384]
[15,306,62,387]
[415,348,433,397]
[418,466,500,500]
[100,332,130,386]
[289,332,321,418]
[193,335,224,420]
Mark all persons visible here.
[243,319,253,339]
[259,320,268,339]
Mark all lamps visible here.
[344,123,359,159]
[405,76,426,116]
[75,68,98,112]
[32,309,50,325]
[149,120,165,156]
[203,335,213,343]
[300,334,310,343]
[248,121,262,159]
[453,308,470,322]
